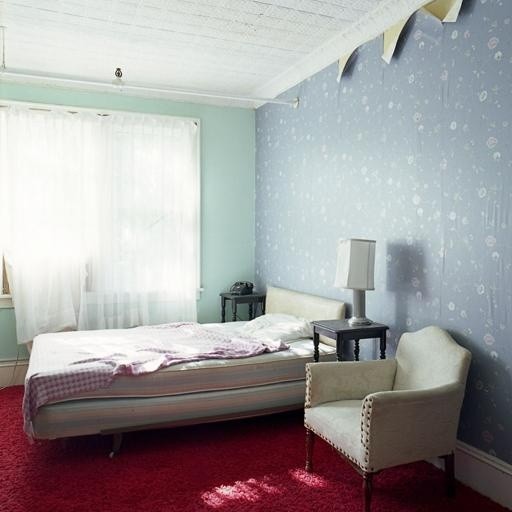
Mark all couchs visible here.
[303,324,472,512]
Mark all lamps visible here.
[335,238,376,325]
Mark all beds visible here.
[25,285,347,455]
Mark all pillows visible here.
[243,311,315,342]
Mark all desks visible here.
[310,317,389,362]
[220,292,266,321]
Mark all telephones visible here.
[230,281,254,295]
